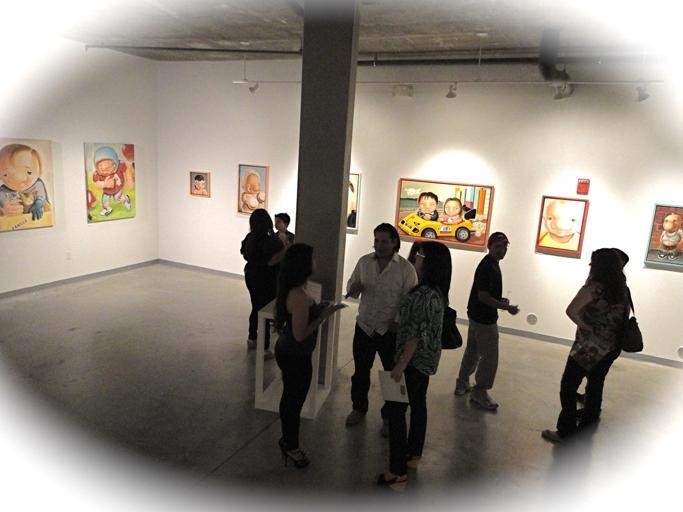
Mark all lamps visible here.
[636,85,650,102]
[551,85,566,100]
[446,84,457,98]
[248,83,259,94]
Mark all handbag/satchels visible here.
[441,307,462,349]
[622,317,643,352]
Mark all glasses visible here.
[414,251,425,258]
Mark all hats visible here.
[489,232,509,243]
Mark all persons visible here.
[542,248,630,444]
[539,199,580,250]
[417,193,438,221]
[455,232,520,410]
[574,249,629,418]
[193,175,207,195]
[93,147,131,216]
[242,171,265,211]
[347,224,419,437]
[275,244,338,468]
[240,209,288,361]
[440,198,463,225]
[269,213,295,325]
[657,213,683,261]
[375,242,451,485]
[0,144,51,221]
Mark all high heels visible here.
[375,455,421,494]
[278,435,311,468]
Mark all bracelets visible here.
[319,315,326,324]
[579,321,585,330]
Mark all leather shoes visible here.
[382,418,389,437]
[346,410,367,427]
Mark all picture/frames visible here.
[394,178,495,253]
[238,164,270,215]
[534,195,590,259]
[345,173,361,235]
[644,204,683,272]
[189,171,211,198]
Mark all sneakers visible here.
[455,383,499,408]
[542,393,601,445]
[247,340,273,360]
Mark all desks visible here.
[255,295,336,419]
